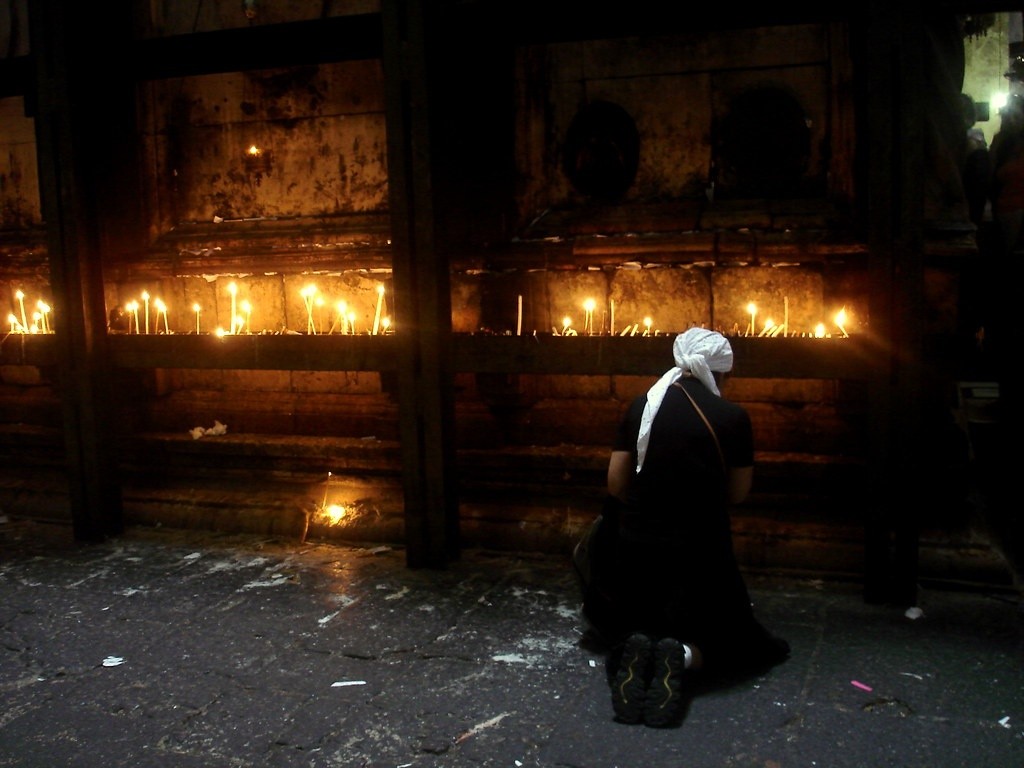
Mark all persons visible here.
[572,327,792,730]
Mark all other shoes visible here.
[641,636,687,728]
[612,634,654,725]
